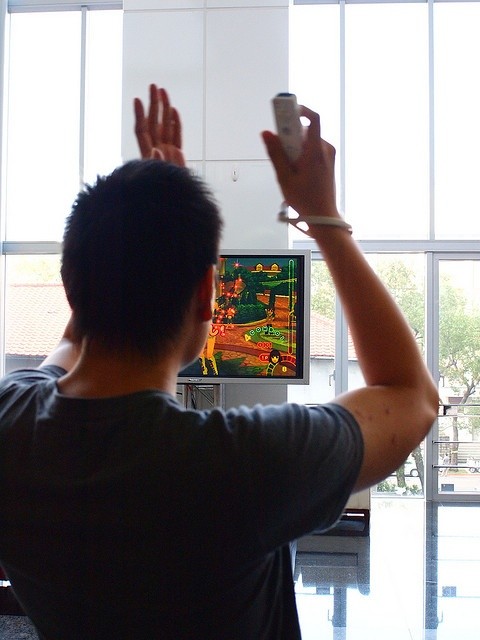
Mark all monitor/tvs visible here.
[177,248,309,386]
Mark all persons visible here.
[0,82,441,639]
